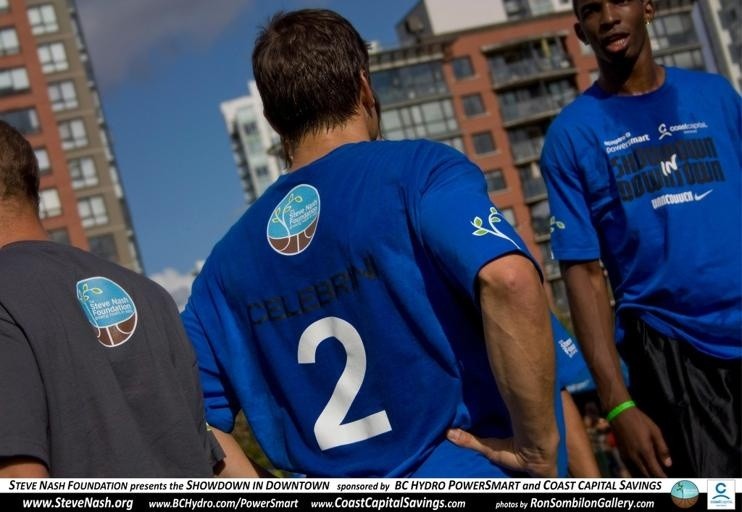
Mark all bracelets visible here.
[607,400,636,422]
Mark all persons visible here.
[548,309,608,477]
[582,414,634,477]
[1,120,227,478]
[182,9,560,477]
[538,0,742,478]
